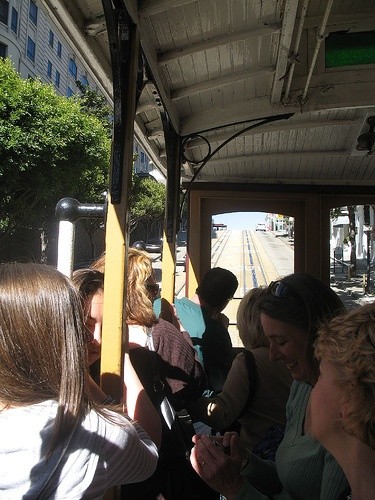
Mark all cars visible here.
[254,223,266,231]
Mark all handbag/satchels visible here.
[163,409,194,461]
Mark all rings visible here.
[200,461,206,468]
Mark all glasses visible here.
[268,281,302,302]
[145,283,159,295]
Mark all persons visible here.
[308,301,375,500]
[0,263,159,500]
[91,249,207,409]
[190,274,352,500]
[153,266,239,394]
[187,287,294,462]
[70,268,161,500]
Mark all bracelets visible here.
[100,395,113,407]
